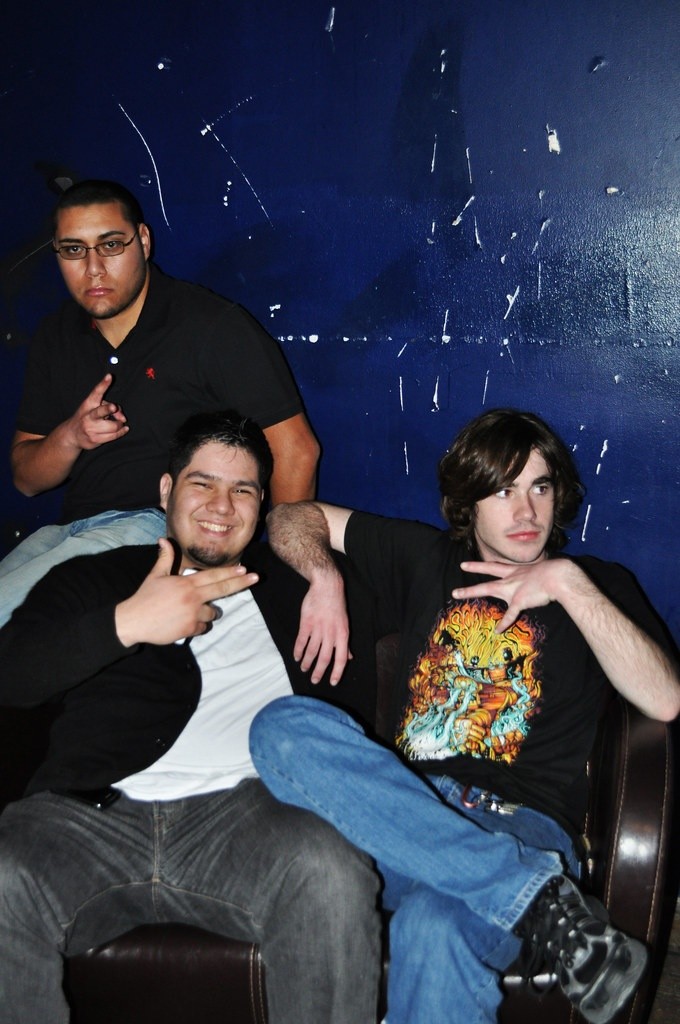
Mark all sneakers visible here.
[512,873,647,1024]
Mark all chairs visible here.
[59,626,677,1024]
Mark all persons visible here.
[249,408,680,1024]
[0,404,386,1024]
[9,177,324,526]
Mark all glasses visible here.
[52,232,139,260]
